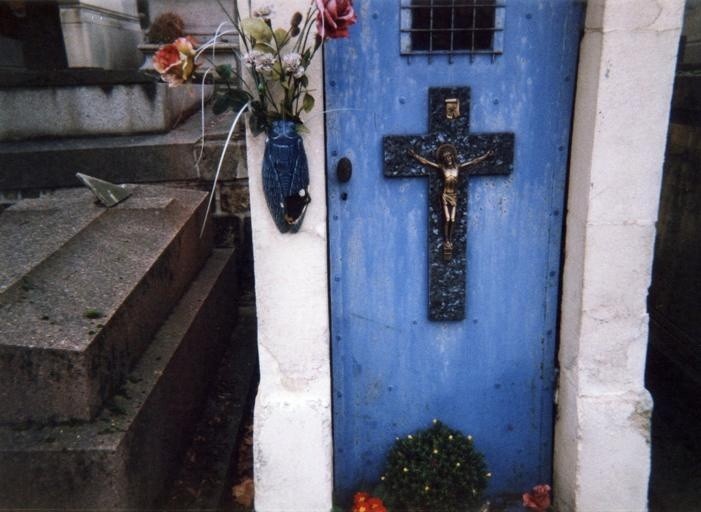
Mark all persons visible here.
[410,146,494,253]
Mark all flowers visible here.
[152,0,357,121]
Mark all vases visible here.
[261,120,310,234]
